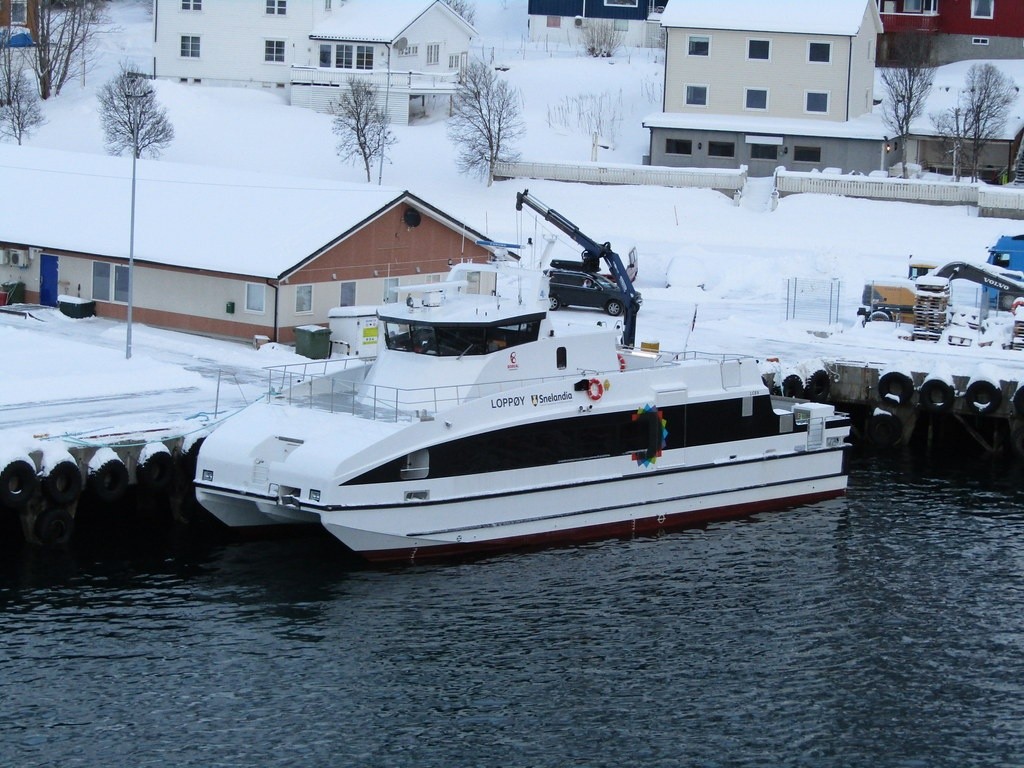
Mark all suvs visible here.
[547,267,642,317]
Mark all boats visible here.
[179,188,851,567]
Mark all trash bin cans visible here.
[293,324,333,362]
[1,281,27,306]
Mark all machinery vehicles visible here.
[859,259,1024,324]
